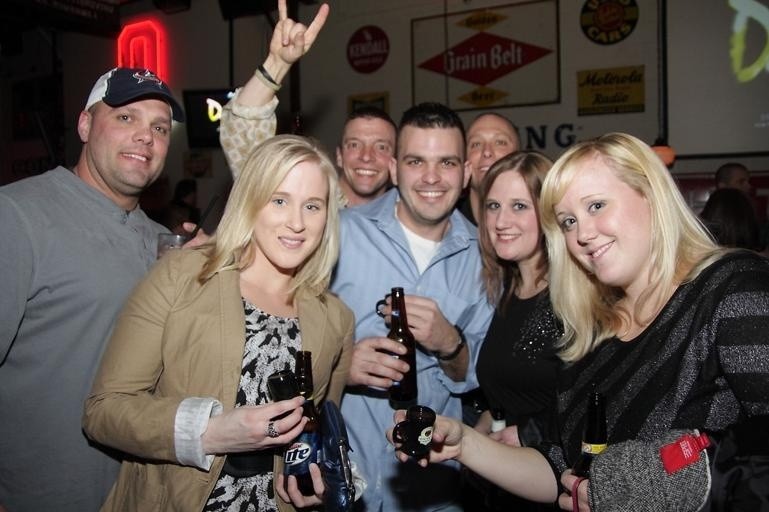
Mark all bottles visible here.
[284,350,319,496]
[570,391,610,478]
[389,286,418,410]
[489,407,508,431]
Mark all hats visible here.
[84,65,186,131]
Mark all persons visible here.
[1,0,769,512]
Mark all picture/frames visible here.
[410,0,563,115]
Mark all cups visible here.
[375,291,391,328]
[267,369,298,403]
[156,232,183,258]
[392,404,436,454]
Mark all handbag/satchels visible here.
[317,398,355,512]
